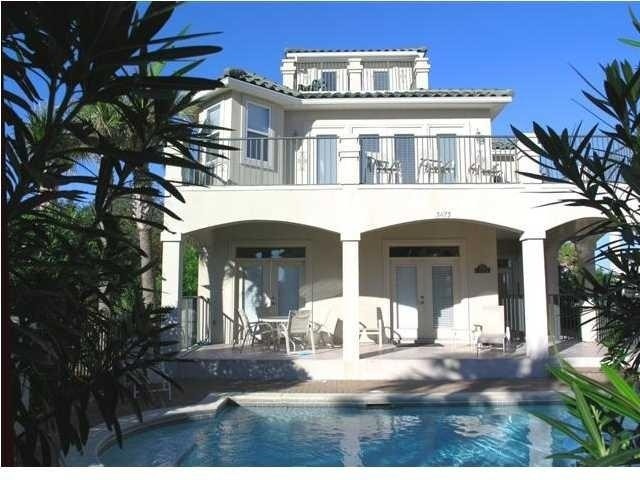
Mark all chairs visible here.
[471,305,511,358]
[129,361,172,401]
[417,158,453,184]
[359,306,382,348]
[232,306,335,355]
[363,150,400,185]
[467,162,501,183]
